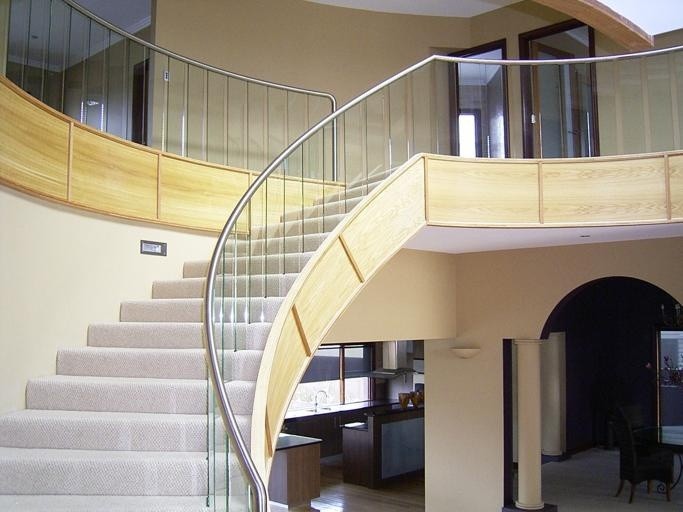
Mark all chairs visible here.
[608,406,674,504]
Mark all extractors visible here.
[365,339,419,379]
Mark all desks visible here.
[632,426,683,494]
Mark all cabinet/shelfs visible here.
[284,399,400,458]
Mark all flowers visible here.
[644,352,682,385]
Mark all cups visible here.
[399,391,421,408]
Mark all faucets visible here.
[314,391,328,406]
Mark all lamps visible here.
[450,349,481,358]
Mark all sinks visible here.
[308,408,332,413]
